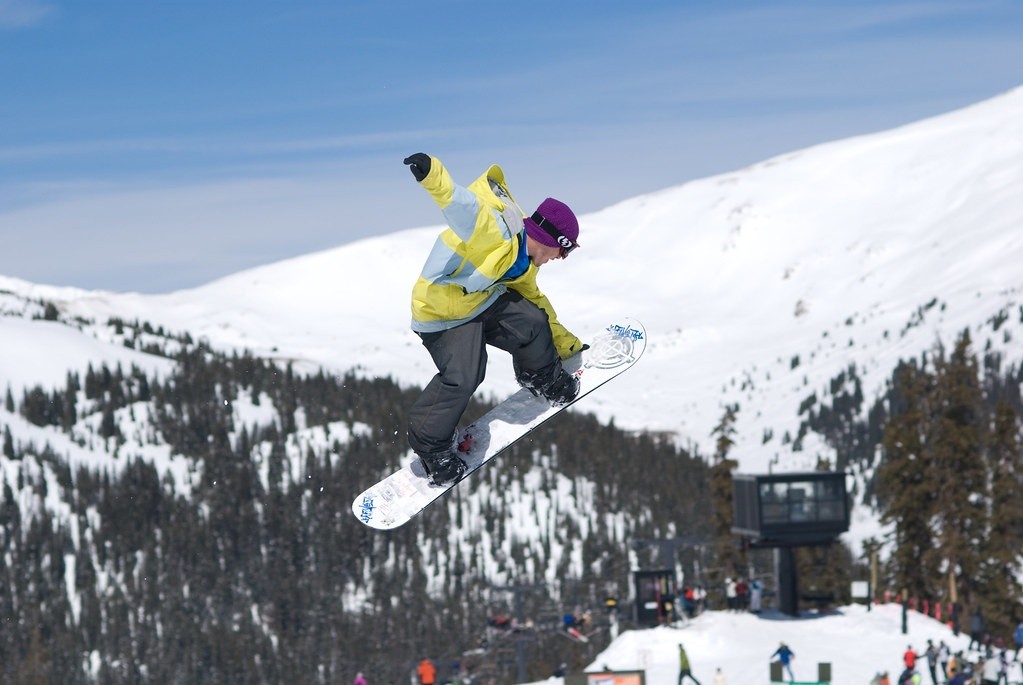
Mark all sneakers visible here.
[525,370,580,404]
[422,452,465,487]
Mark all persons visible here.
[402,150,593,488]
[333,534,1023,685]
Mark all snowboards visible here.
[350,314,648,532]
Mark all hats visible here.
[523,198,579,245]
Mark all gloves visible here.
[402,153,432,182]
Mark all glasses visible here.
[532,213,578,258]
